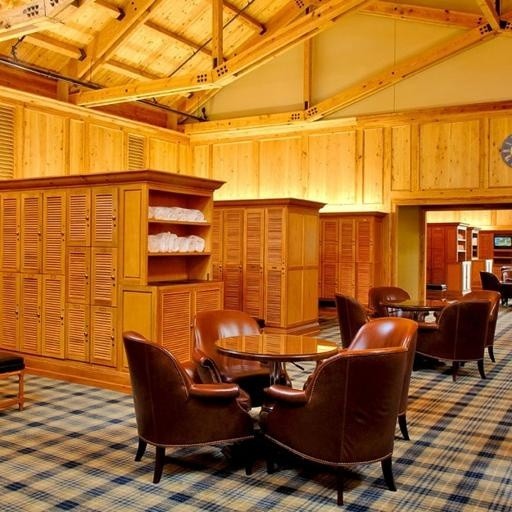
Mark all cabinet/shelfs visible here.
[427,223,492,300]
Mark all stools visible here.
[0,352,25,412]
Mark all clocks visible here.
[502,134,512,168]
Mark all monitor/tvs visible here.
[494,235,512,248]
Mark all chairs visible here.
[415,300,492,382]
[259,347,408,506]
[347,317,418,441]
[480,266,512,306]
[434,290,501,366]
[368,287,429,322]
[122,332,259,484]
